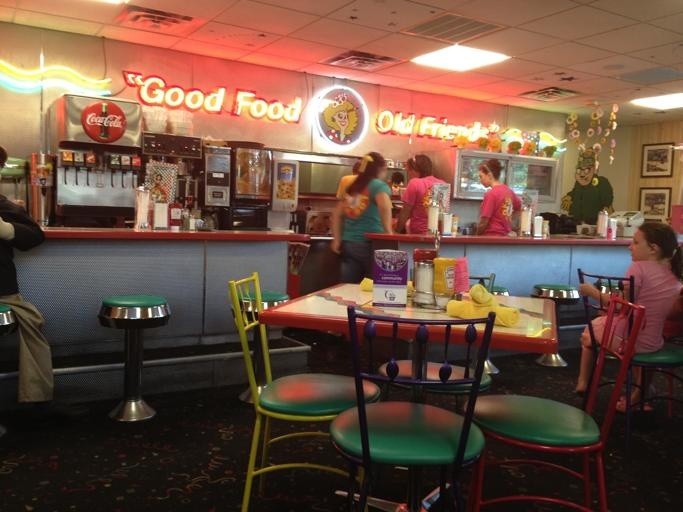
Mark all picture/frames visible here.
[641,142,674,176]
[639,187,672,222]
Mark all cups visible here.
[147,105,196,138]
[462,228,471,234]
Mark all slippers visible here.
[616,396,656,413]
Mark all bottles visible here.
[597,210,617,240]
[520,207,551,238]
[132,184,201,233]
[413,248,469,308]
[100,103,111,139]
[426,200,459,235]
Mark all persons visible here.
[328,152,394,285]
[0,146,60,406]
[334,160,363,205]
[433,193,446,209]
[394,153,447,233]
[386,170,405,196]
[571,220,683,412]
[473,157,522,237]
[631,246,682,412]
[150,172,167,203]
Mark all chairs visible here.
[330,306,497,512]
[577,267,682,439]
[463,292,646,511]
[229,270,380,512]
[376,273,497,411]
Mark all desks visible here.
[258,280,560,512]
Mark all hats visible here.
[576,151,595,167]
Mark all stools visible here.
[97,295,171,424]
[468,282,621,374]
[232,291,292,406]
[1,305,15,437]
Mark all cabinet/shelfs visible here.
[439,148,561,204]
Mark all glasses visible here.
[576,168,591,175]
